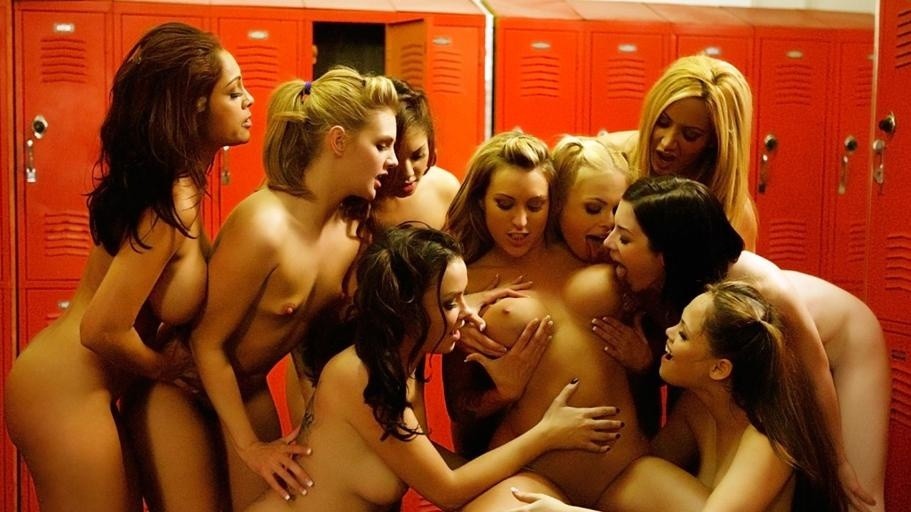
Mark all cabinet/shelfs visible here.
[0,0,910,511]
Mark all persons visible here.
[6,22,891,512]
